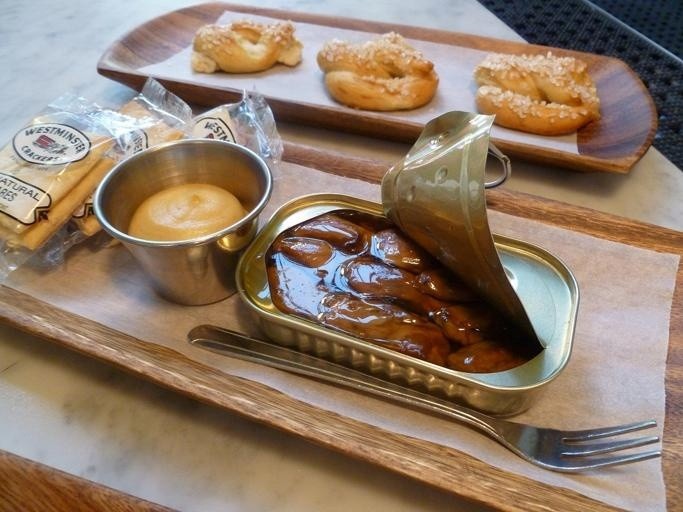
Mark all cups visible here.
[92,139,272,306]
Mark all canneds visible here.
[235,111,579,419]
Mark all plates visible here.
[0,106,683,510]
[95,2,658,176]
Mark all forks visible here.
[186,323,661,472]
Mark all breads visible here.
[474,52,600,134]
[129,180,244,241]
[317,32,438,110]
[190,19,303,75]
